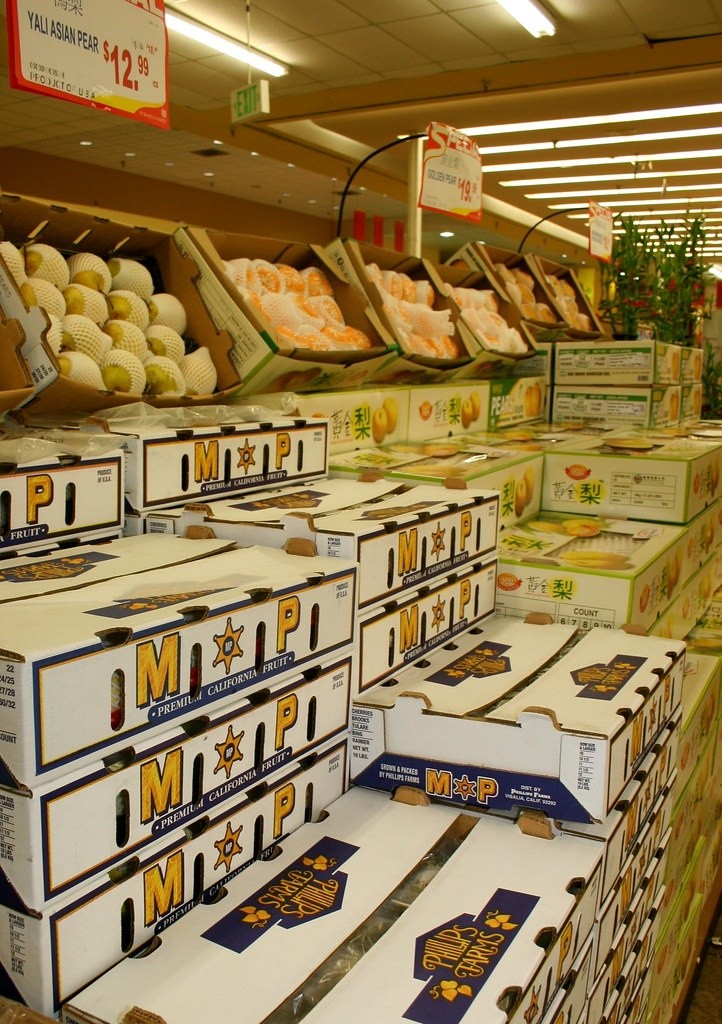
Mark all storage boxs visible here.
[0,192,722,1024]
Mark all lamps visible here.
[496,0,560,40]
[165,6,290,79]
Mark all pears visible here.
[223,261,589,353]
[369,353,722,618]
[0,242,218,395]
